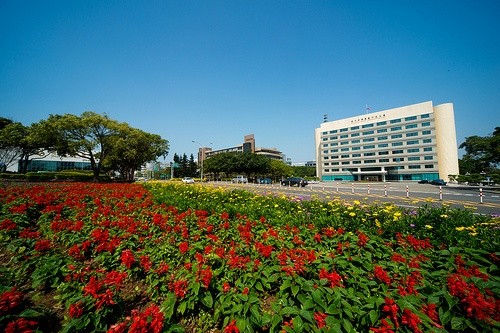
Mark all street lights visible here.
[192,141,203,182]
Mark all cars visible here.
[135,177,148,182]
[418,180,428,183]
[283,178,308,187]
[430,179,447,186]
[232,175,247,182]
[181,176,194,183]
[257,178,272,183]
